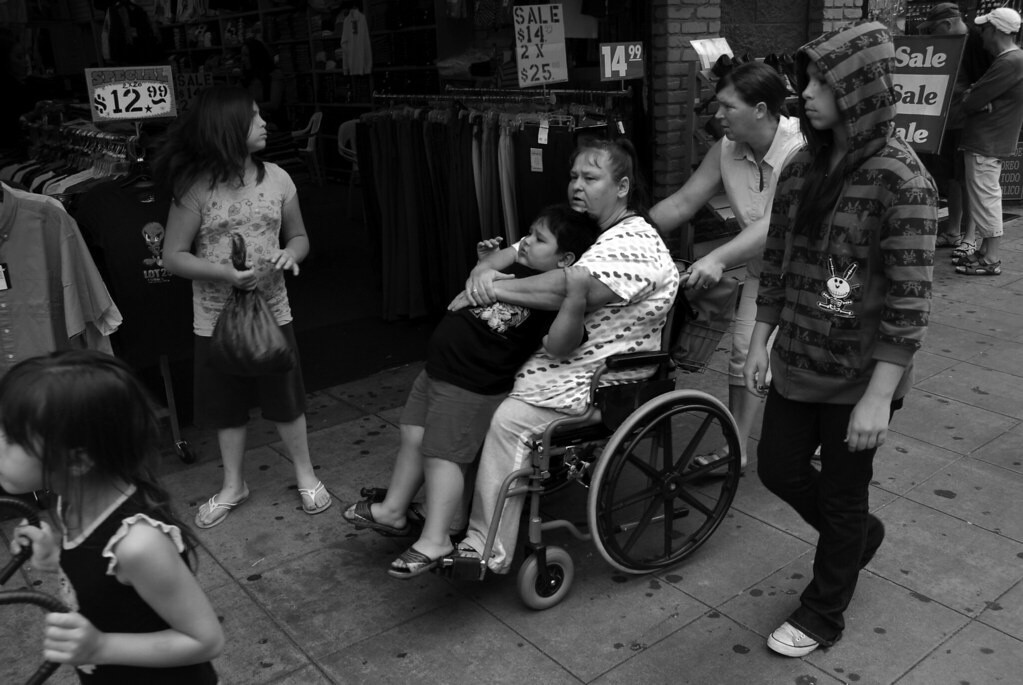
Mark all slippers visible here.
[195,494,249,529]
[298,480,331,515]
[340,500,411,535]
[387,546,454,579]
[689,450,745,477]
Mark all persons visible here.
[647,63,807,474]
[161,91,332,529]
[916,3,1023,276]
[448,138,680,558]
[743,23,936,657]
[341,202,602,579]
[0,348,226,685]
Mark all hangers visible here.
[365,86,626,132]
[13,116,151,195]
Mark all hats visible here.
[973,7,1021,34]
[916,3,961,29]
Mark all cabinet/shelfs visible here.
[153,0,474,185]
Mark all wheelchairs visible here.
[359,259,741,608]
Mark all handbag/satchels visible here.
[208,232,289,382]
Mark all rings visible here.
[473,290,477,292]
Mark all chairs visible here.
[260,110,325,180]
[335,115,374,230]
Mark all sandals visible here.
[953,240,977,256]
[935,232,962,247]
[952,250,1002,274]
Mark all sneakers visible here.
[766,621,819,657]
